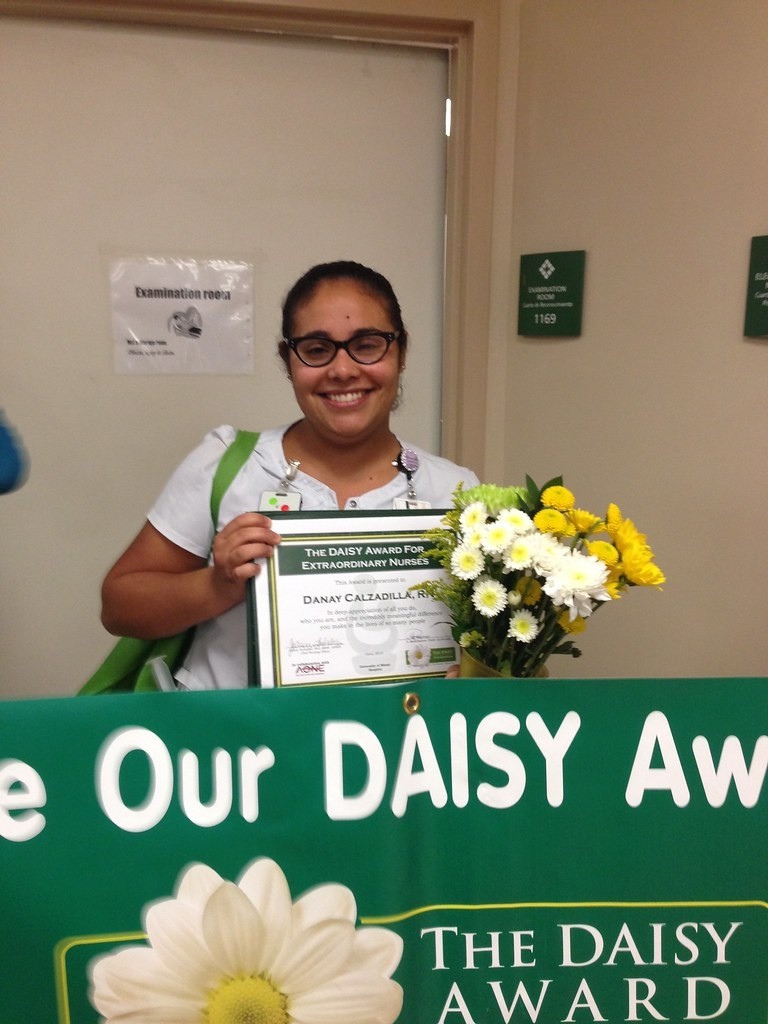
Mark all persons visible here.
[98,260,480,691]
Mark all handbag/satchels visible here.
[75,429,258,698]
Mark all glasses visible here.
[280,318,401,368]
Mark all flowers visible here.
[407,475,667,677]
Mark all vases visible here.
[444,646,550,678]
